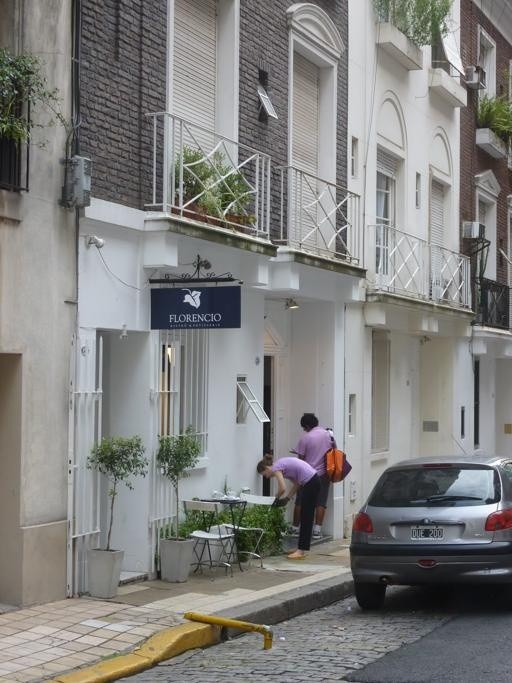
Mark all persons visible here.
[257,413,336,559]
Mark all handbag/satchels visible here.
[326,448,353,483]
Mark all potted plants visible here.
[171,144,256,233]
[156,423,195,583]
[85,434,149,599]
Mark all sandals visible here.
[287,549,305,561]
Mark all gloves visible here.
[272,496,290,507]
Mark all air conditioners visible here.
[466,66,487,90]
[462,221,485,239]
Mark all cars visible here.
[348,430,511,610]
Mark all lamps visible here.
[285,297,299,310]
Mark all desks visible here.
[197,496,247,574]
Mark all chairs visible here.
[224,492,276,569]
[181,499,233,577]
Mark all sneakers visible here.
[289,527,299,537]
[312,530,322,540]
